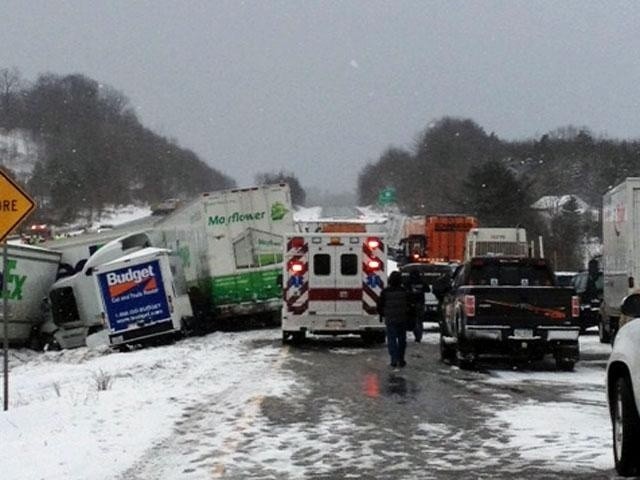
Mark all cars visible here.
[605,292,640,476]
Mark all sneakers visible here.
[391,360,406,367]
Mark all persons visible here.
[377,270,414,368]
[403,268,431,342]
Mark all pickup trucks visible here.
[438,256,581,370]
